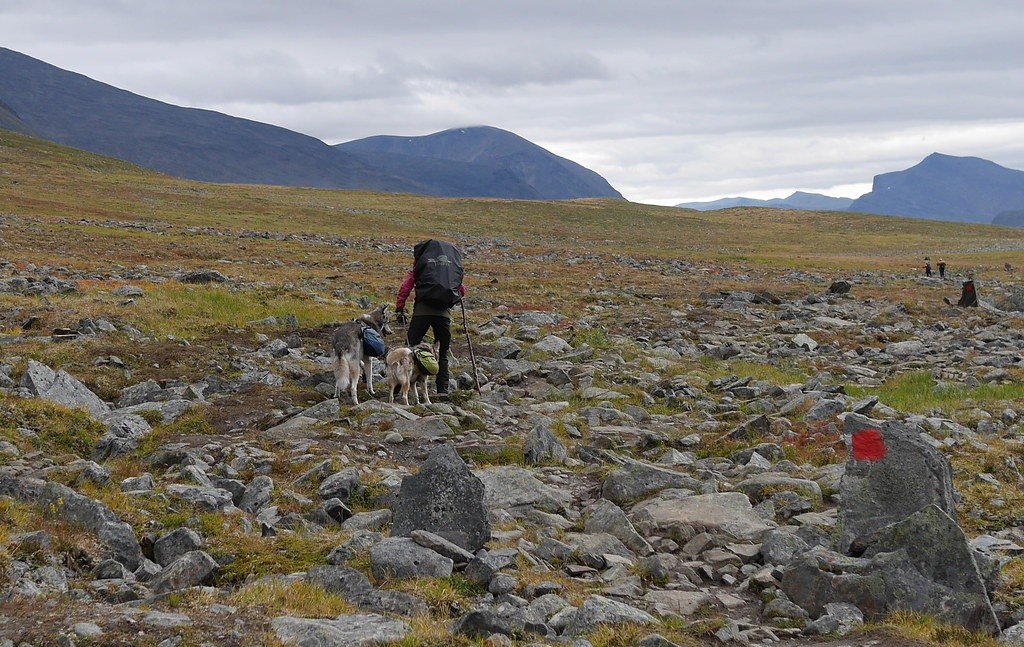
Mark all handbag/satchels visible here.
[413,347,439,376]
[362,326,387,358]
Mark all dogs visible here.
[332,303,392,406]
[386,340,442,406]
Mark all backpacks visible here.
[414,239,463,308]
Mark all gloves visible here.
[395,309,408,324]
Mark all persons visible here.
[936,258,946,277]
[393,254,466,396]
[924,262,931,277]
